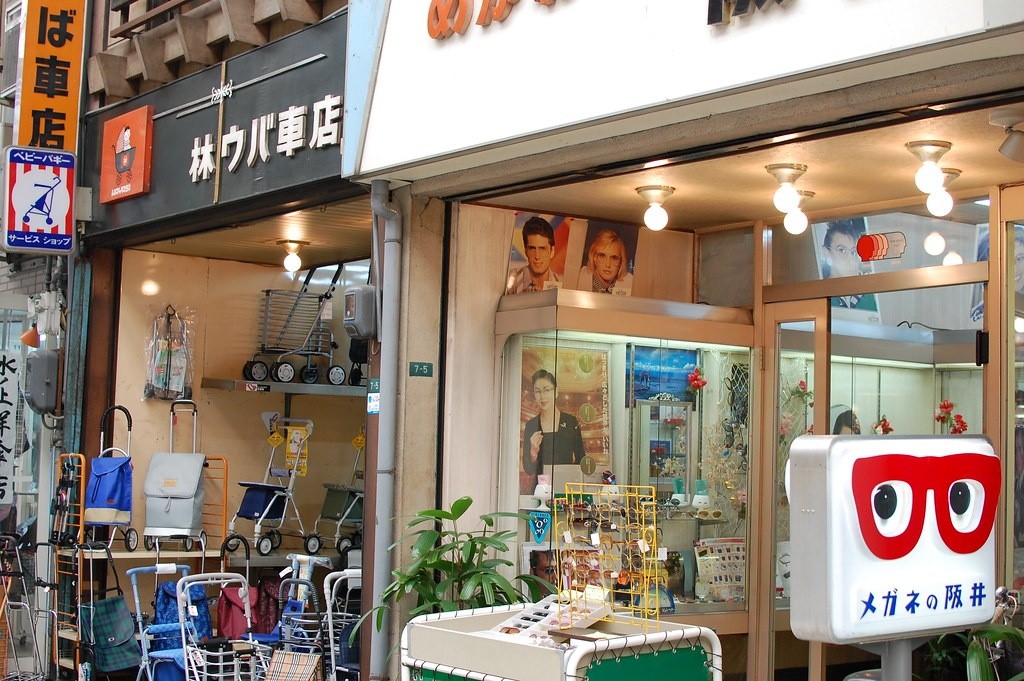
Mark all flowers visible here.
[782,381,805,410]
[936,400,967,435]
[870,414,894,435]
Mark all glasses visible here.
[697,509,724,521]
[824,245,860,259]
[500,495,696,651]
[595,252,623,267]
[532,566,558,575]
[534,388,554,395]
[723,377,748,511]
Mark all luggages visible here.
[144,314,193,402]
[143,398,207,551]
[65,404,137,552]
[217,534,264,643]
[71,542,148,681]
[264,579,327,681]
[124,564,213,681]
[257,576,299,634]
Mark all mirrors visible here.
[635,399,692,506]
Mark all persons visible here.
[505,217,559,294]
[530,551,558,601]
[832,410,861,437]
[821,223,876,312]
[522,369,586,490]
[970,226,1024,322]
[576,229,633,298]
[561,551,575,591]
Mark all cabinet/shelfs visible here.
[201,377,367,567]
[50,453,227,681]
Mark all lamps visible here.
[276,240,310,272]
[907,140,952,193]
[783,190,815,234]
[635,186,675,231]
[926,168,961,217]
[998,130,1024,163]
[765,163,808,213]
